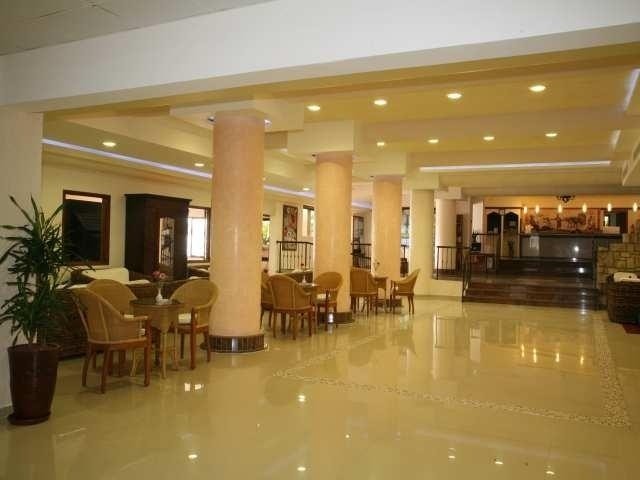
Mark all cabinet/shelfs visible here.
[606,273,640,325]
[123,193,193,334]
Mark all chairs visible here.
[261,267,421,342]
[69,267,219,392]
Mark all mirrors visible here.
[61,189,112,266]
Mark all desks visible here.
[129,297,184,379]
[298,282,320,333]
[374,276,389,313]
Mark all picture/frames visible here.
[282,205,299,251]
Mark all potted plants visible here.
[0,193,97,428]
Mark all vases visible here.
[149,270,167,303]
